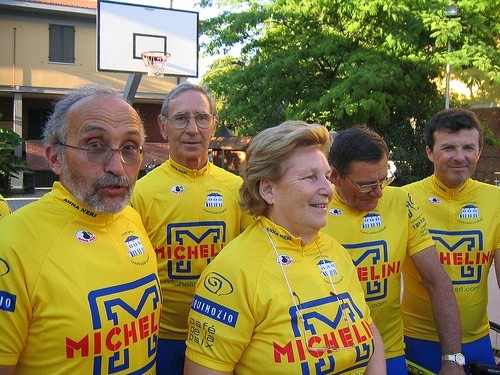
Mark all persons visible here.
[0,86,165,375]
[0,195,12,218]
[180,120,389,375]
[310,127,468,375]
[129,82,258,375]
[205,149,247,171]
[398,107,500,375]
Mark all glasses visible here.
[164,112,216,129]
[345,168,396,193]
[63,142,144,166]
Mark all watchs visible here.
[441,351,465,366]
